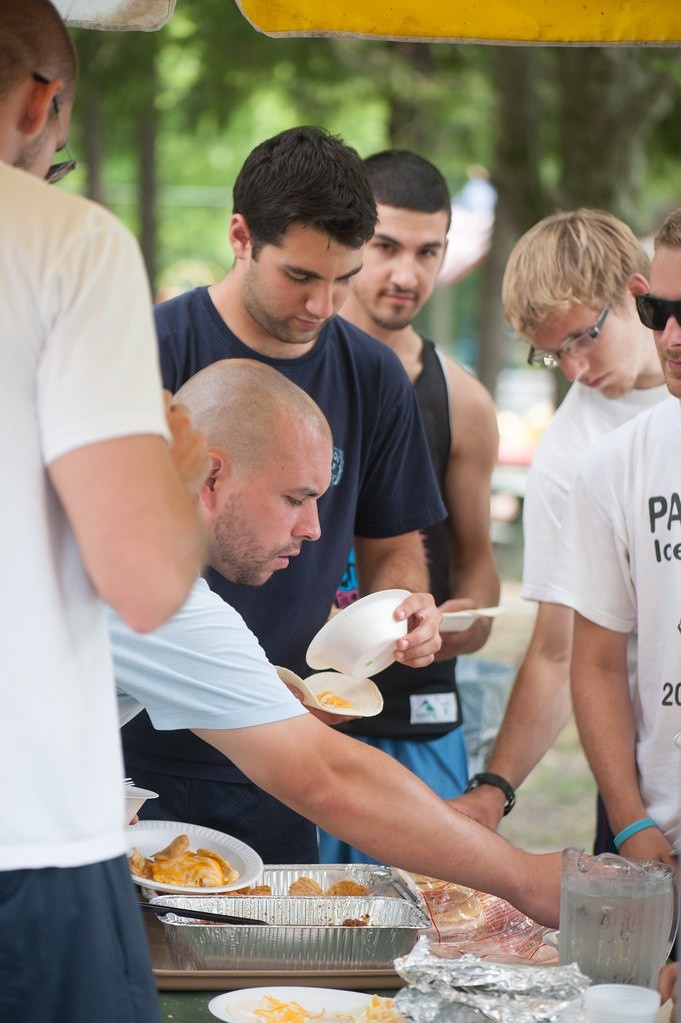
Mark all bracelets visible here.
[464,772,517,816]
[613,817,657,852]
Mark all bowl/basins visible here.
[306,589,413,679]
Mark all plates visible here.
[124,787,159,824]
[124,820,264,895]
[208,986,387,1023]
[438,612,484,633]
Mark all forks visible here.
[124,777,135,789]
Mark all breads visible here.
[414,874,482,923]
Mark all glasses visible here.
[527,298,615,370]
[636,292,681,331]
[29,71,78,187]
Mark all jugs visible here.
[557,847,680,991]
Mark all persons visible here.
[116,122,444,873]
[438,209,673,857]
[311,144,504,866]
[109,358,626,933]
[0,0,209,1022]
[569,209,681,1019]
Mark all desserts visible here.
[128,834,241,888]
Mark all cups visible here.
[583,983,663,1023]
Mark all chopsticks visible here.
[137,901,267,925]
